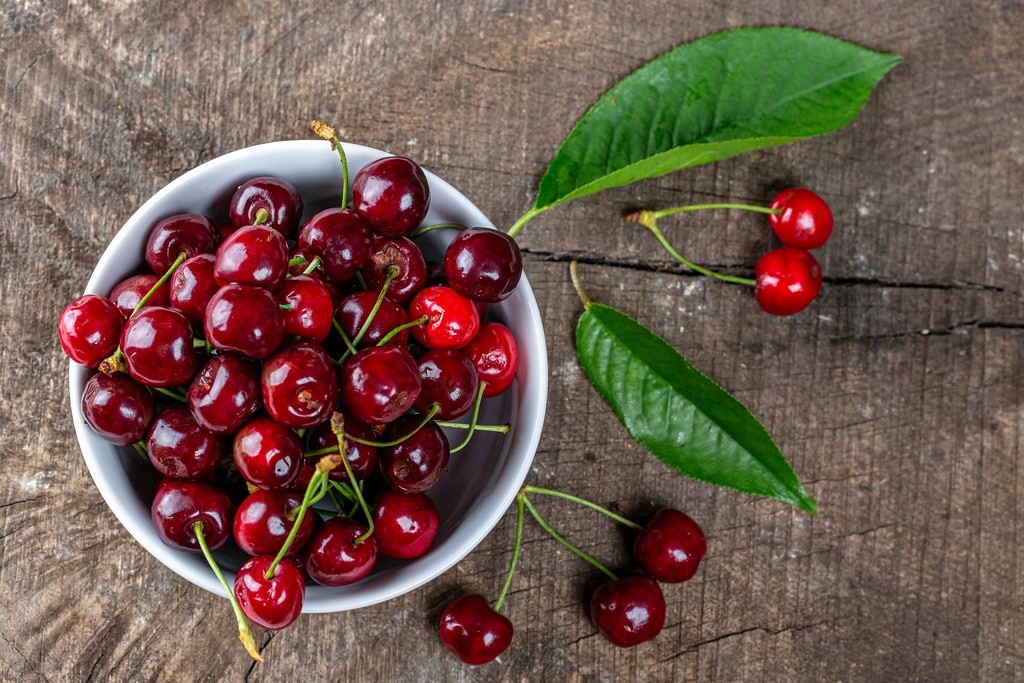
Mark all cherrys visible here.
[53,119,524,633]
[440,485,707,665]
[626,187,834,317]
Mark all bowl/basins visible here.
[66,135,549,619]
